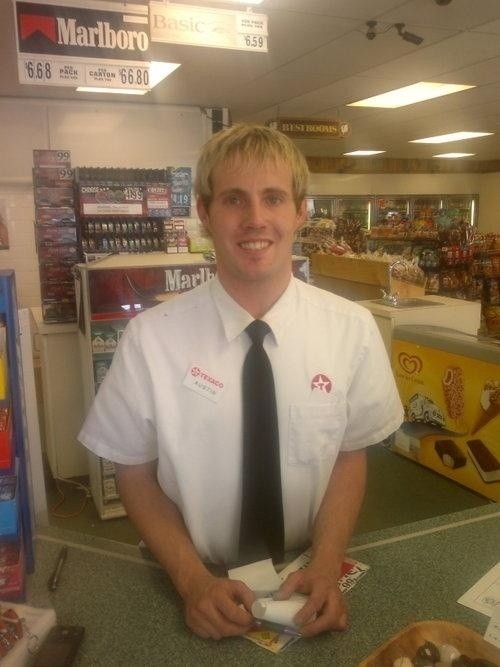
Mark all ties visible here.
[239,320,284,568]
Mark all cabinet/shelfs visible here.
[30,148,500,524]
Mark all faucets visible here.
[389,261,408,296]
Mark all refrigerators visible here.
[381,323,500,503]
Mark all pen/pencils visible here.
[252,618,303,637]
[48,544,67,591]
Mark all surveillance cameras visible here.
[364,26,376,40]
[402,30,425,45]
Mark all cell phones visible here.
[32,624,84,666]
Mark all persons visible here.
[74,118,406,643]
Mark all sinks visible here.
[370,296,447,308]
